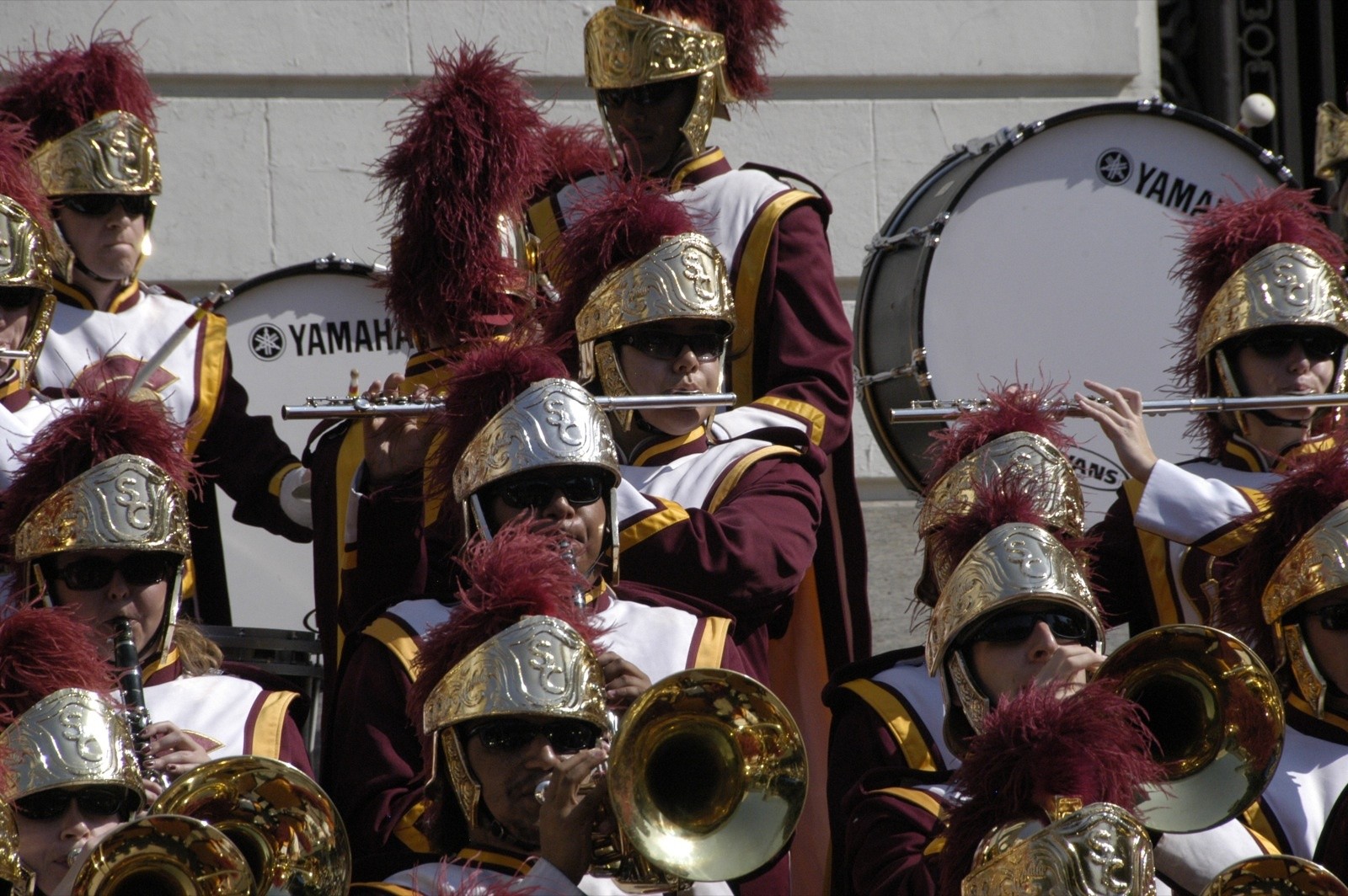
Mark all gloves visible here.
[282,464,380,547]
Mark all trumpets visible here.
[1066,623,1286,835]
[67,753,352,896]
[1199,853,1348,896]
[531,667,811,896]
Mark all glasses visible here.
[970,605,1101,646]
[466,718,623,762]
[11,785,131,822]
[604,321,747,368]
[1227,324,1346,361]
[0,283,39,312]
[597,81,679,109]
[1300,603,1348,633]
[57,192,155,218]
[495,471,626,511]
[51,552,175,595]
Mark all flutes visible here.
[892,392,1348,424]
[111,621,163,813]
[282,386,739,419]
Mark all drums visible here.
[191,255,427,635]
[854,97,1310,519]
[183,624,322,768]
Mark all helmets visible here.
[1163,176,1348,437]
[369,41,612,331]
[415,342,624,553]
[1310,101,1348,182]
[917,670,1166,896]
[414,508,613,826]
[0,12,164,283]
[0,604,149,896]
[535,166,743,430]
[916,459,1114,729]
[1,335,218,668]
[579,0,792,159]
[1209,424,1346,719]
[912,377,1093,612]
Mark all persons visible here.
[293,0,872,896]
[822,179,1348,896]
[0,34,363,896]
